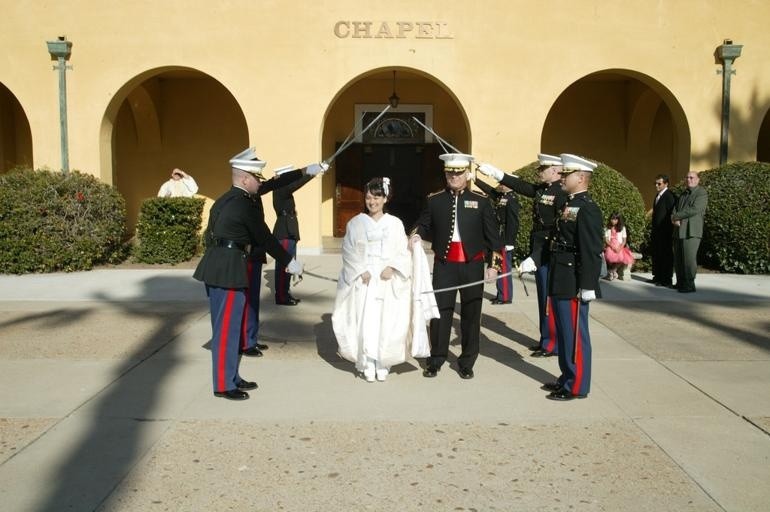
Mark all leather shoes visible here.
[255,344,268,350]
[276,297,297,306]
[213,388,249,401]
[543,380,564,392]
[531,350,558,358]
[647,276,696,292]
[492,299,512,304]
[423,364,437,377]
[549,387,587,400]
[459,367,474,379]
[242,348,263,357]
[235,379,258,390]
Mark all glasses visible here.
[654,181,665,186]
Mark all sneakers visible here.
[605,272,619,281]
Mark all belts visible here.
[532,222,554,232]
[549,241,580,254]
[277,208,296,217]
[208,238,254,257]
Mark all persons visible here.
[193,156,308,403]
[469,152,607,400]
[602,210,634,281]
[329,176,442,384]
[157,167,200,200]
[643,171,709,292]
[271,161,330,306]
[407,151,501,380]
[228,145,269,358]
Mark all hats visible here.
[536,152,597,176]
[229,146,266,181]
[438,153,474,175]
[273,165,294,175]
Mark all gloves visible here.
[477,163,504,183]
[286,257,304,276]
[576,289,596,303]
[306,164,325,177]
[505,245,514,252]
[518,256,537,273]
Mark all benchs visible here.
[601,249,646,282]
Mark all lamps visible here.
[389,70,398,112]
